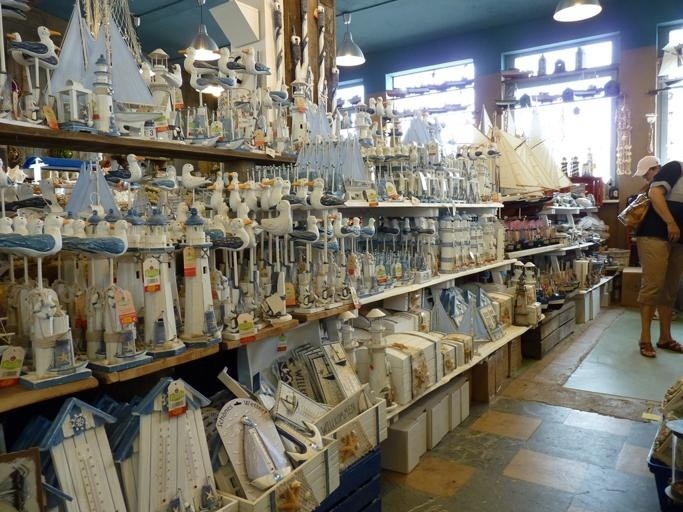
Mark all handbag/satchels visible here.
[616,193,651,228]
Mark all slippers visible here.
[656,340,682,353]
[638,341,656,357]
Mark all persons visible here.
[633,156,678,321]
[636,161,683,358]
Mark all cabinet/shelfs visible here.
[0,121,614,512]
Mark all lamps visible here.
[183,0,219,63]
[553,1,603,23]
[336,11,366,68]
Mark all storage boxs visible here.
[621,266,645,306]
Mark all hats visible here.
[631,155,661,178]
